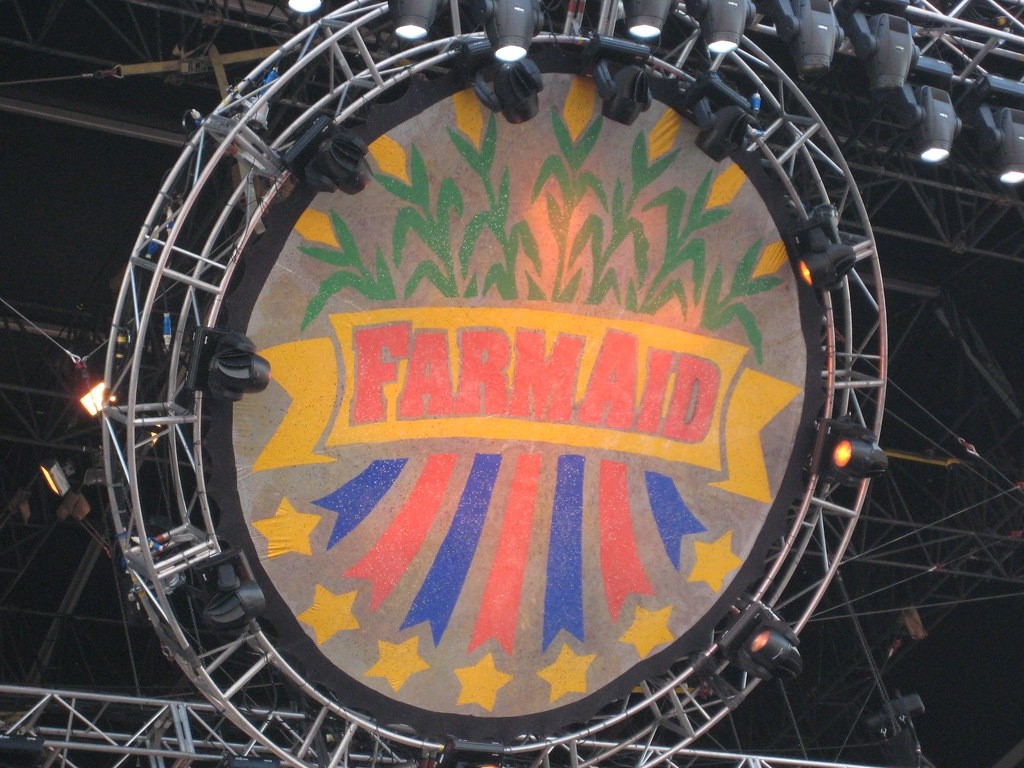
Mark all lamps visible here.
[818,415,888,487]
[395,1,438,40]
[718,600,802,681]
[867,694,924,729]
[185,544,266,631]
[0,733,504,768]
[891,56,962,163]
[695,105,751,163]
[774,0,845,78]
[39,366,117,522]
[471,63,544,124]
[624,0,670,37]
[595,61,653,127]
[797,209,858,288]
[971,73,1024,184]
[284,116,372,196]
[471,0,544,62]
[836,0,920,93]
[187,326,271,402]
[697,0,756,53]
